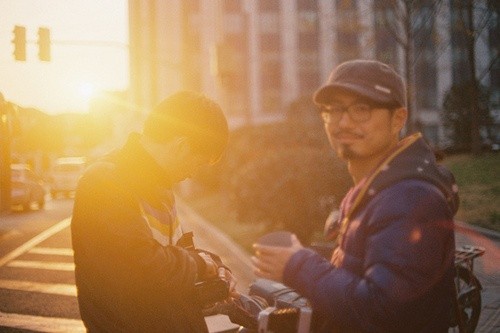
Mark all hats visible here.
[313,60,406,108]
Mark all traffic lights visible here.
[13,25,28,63]
[37,27,52,63]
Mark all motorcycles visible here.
[221,244,487,332]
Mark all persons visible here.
[71,90,240,333]
[251,59,461,333]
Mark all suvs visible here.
[49,157,87,199]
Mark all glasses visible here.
[319,101,388,124]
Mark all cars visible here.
[11,163,46,212]
